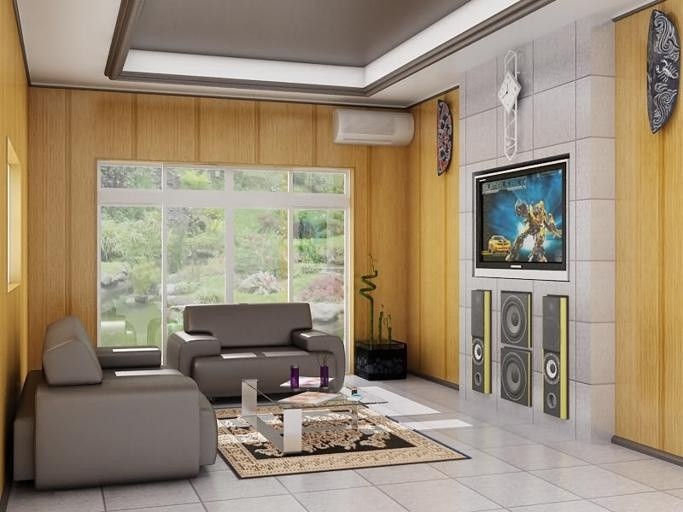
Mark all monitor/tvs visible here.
[474,159,569,281]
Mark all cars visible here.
[486,235,512,254]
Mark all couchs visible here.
[12,312,217,492]
[167,301,346,403]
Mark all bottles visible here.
[291,365,299,391]
[320,366,328,389]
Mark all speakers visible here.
[542,296,568,420]
[500,293,532,348]
[501,347,532,407]
[471,291,490,393]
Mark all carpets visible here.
[215,399,472,481]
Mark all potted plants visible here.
[355,255,407,381]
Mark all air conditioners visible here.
[332,108,415,145]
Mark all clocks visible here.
[497,71,521,112]
